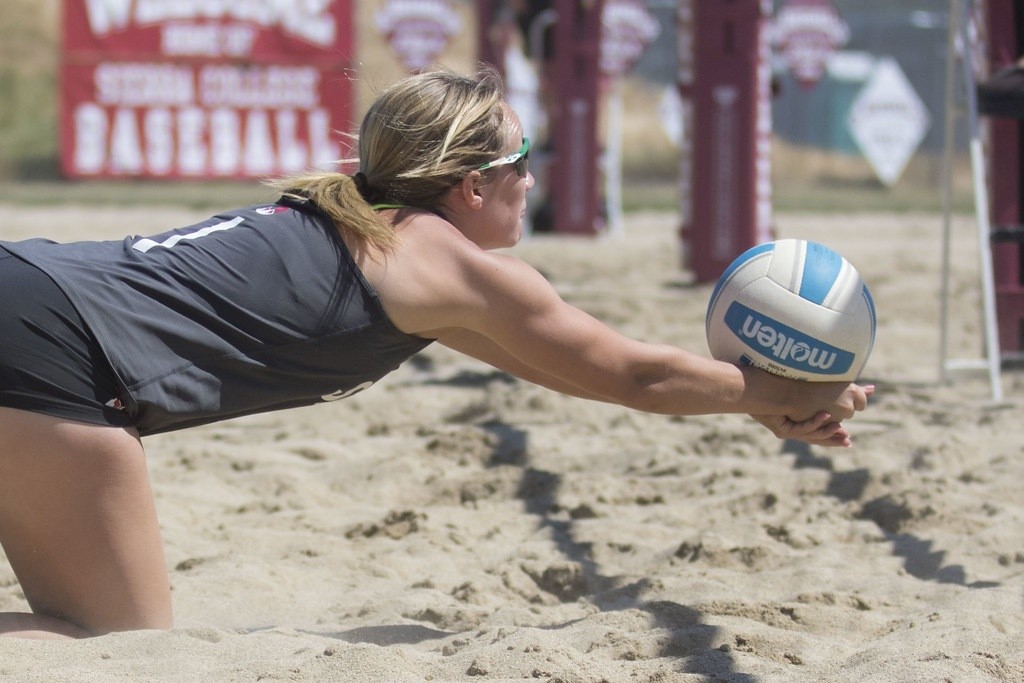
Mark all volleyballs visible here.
[705,236,879,383]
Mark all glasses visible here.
[470,135,532,180]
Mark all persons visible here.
[0,60,875,641]
